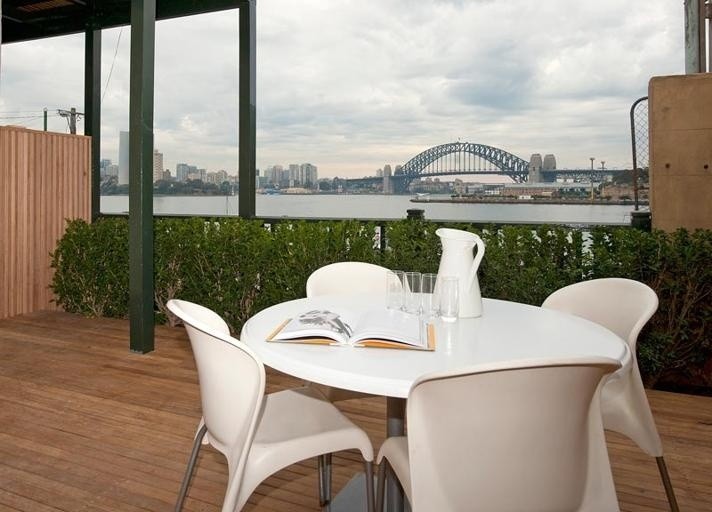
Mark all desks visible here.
[238,292,632,512]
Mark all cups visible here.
[385,270,459,323]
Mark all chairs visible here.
[305,260,404,512]
[541,276,682,510]
[163,298,375,512]
[375,356,624,511]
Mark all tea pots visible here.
[432,228,485,318]
